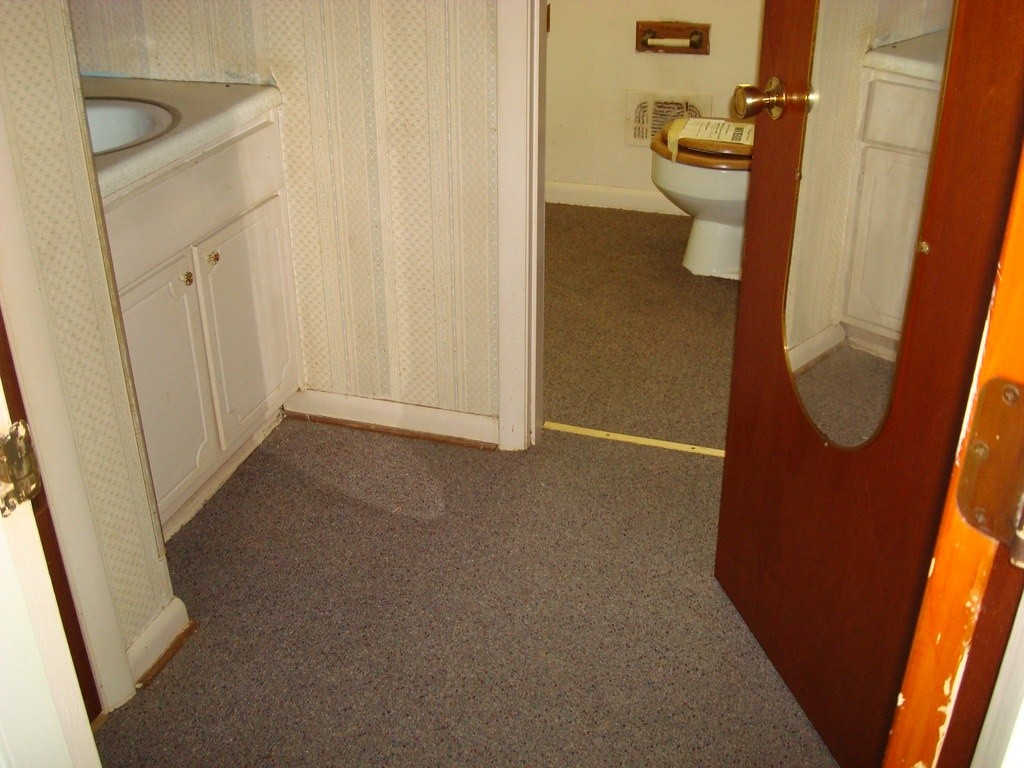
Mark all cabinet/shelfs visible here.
[839,67,943,363]
[101,120,301,544]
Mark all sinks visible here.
[84,93,180,156]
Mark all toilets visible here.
[648,115,756,281]
